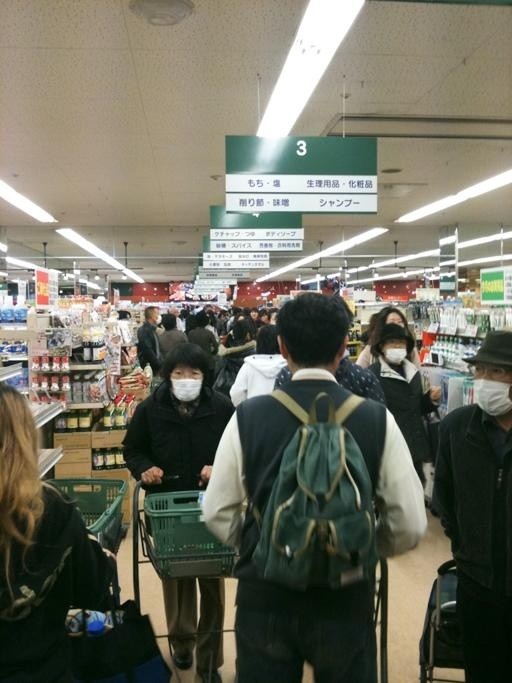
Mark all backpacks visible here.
[248,389,378,590]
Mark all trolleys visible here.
[41,474,132,634]
[418,554,466,683]
[130,471,243,637]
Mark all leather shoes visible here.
[196,665,222,683]
[173,652,193,669]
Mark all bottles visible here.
[429,336,484,370]
[82,324,108,363]
[144,362,153,382]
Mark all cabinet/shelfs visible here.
[1,359,65,480]
[69,363,108,408]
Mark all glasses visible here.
[467,364,512,379]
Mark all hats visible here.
[370,323,414,355]
[462,330,512,368]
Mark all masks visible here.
[383,349,407,366]
[471,379,512,417]
[170,379,203,402]
[156,315,162,325]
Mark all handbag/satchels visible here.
[421,396,441,466]
[69,600,171,683]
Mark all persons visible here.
[427,329,512,682]
[118,343,237,682]
[366,324,440,473]
[356,307,429,392]
[121,303,278,466]
[229,325,287,407]
[1,381,116,681]
[203,292,427,683]
[274,293,386,406]
